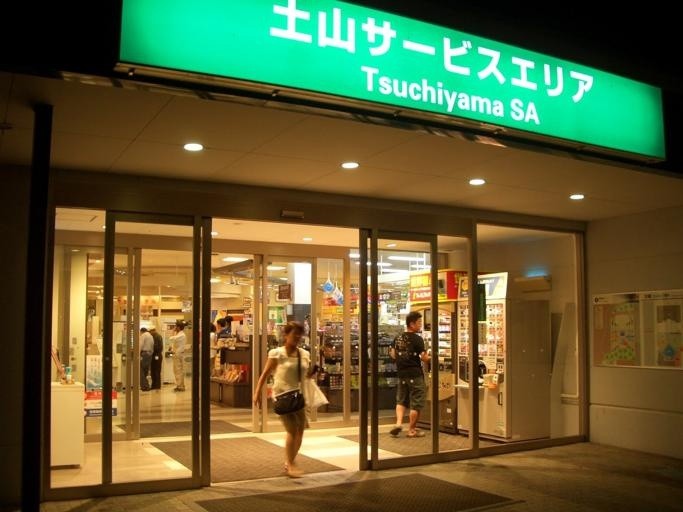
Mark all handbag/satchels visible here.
[272,388,304,414]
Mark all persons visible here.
[148,329,163,389]
[388,311,431,437]
[209,316,233,377]
[254,322,319,478]
[138,328,154,391]
[170,320,185,392]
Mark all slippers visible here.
[407,431,425,438]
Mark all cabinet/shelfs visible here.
[316,325,406,412]
[210,334,252,408]
[456,299,552,443]
[409,302,457,435]
